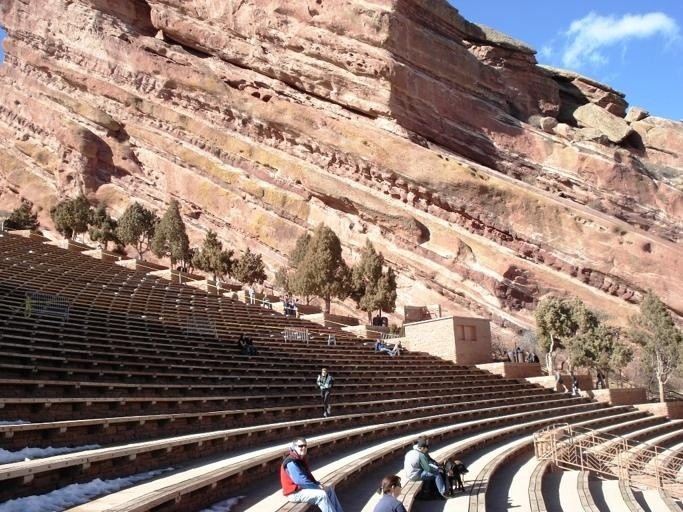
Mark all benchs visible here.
[1,232,683,511]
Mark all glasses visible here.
[297,445,307,448]
[397,482,401,488]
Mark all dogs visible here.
[443,458,469,497]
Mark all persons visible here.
[393,341,405,357]
[554,369,569,393]
[374,474,407,512]
[280,438,343,512]
[239,333,256,357]
[525,350,533,363]
[376,336,396,357]
[596,369,605,390]
[531,352,539,363]
[569,371,580,396]
[405,440,451,499]
[317,368,334,417]
[414,439,440,465]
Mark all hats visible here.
[419,441,430,448]
